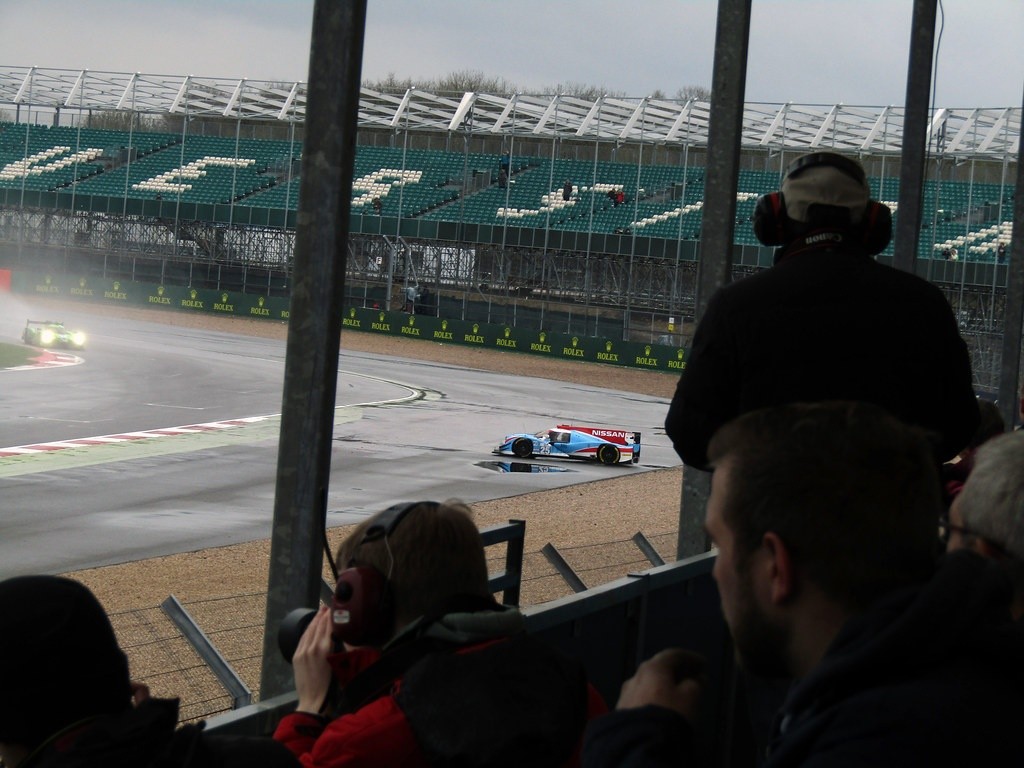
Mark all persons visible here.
[155,193,162,200]
[998,243,1007,264]
[663,150,978,474]
[0,574,304,768]
[563,179,572,201]
[420,286,428,316]
[608,188,625,206]
[941,248,958,261]
[499,148,510,188]
[937,428,1024,560]
[374,198,381,214]
[586,401,1024,768]
[276,501,608,768]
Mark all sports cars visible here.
[489,426,640,466]
[21,319,86,352]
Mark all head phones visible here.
[755,152,894,258]
[329,499,441,647]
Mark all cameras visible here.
[277,607,317,666]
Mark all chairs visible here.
[0,122,1017,266]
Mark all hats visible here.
[781,152,868,226]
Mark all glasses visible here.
[937,516,1006,558]
